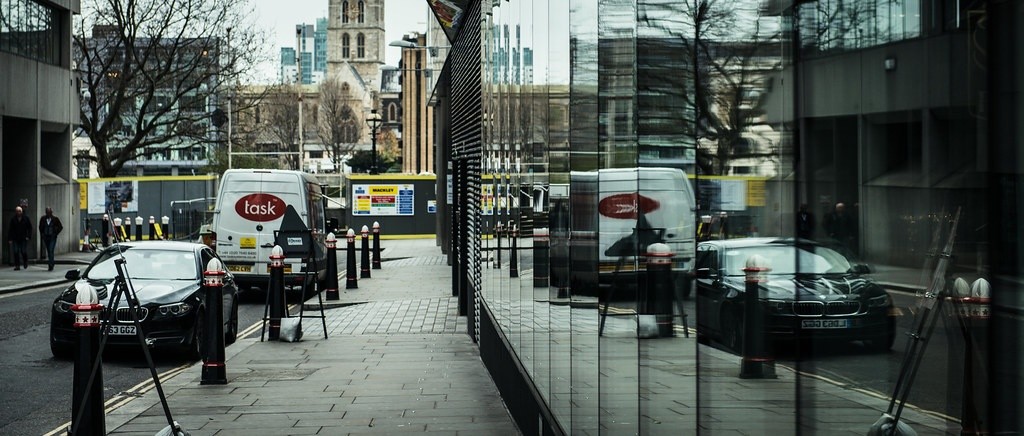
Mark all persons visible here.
[8,206,33,270]
[38,207,63,271]
[824,201,858,259]
[796,202,815,253]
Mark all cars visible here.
[50,241,238,360]
[697,237,895,354]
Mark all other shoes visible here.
[13,267,20,270]
[24,263,27,268]
[48,266,53,271]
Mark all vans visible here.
[212,169,338,299]
[570,167,699,300]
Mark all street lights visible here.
[226,27,232,169]
[366,109,384,175]
[297,29,304,169]
